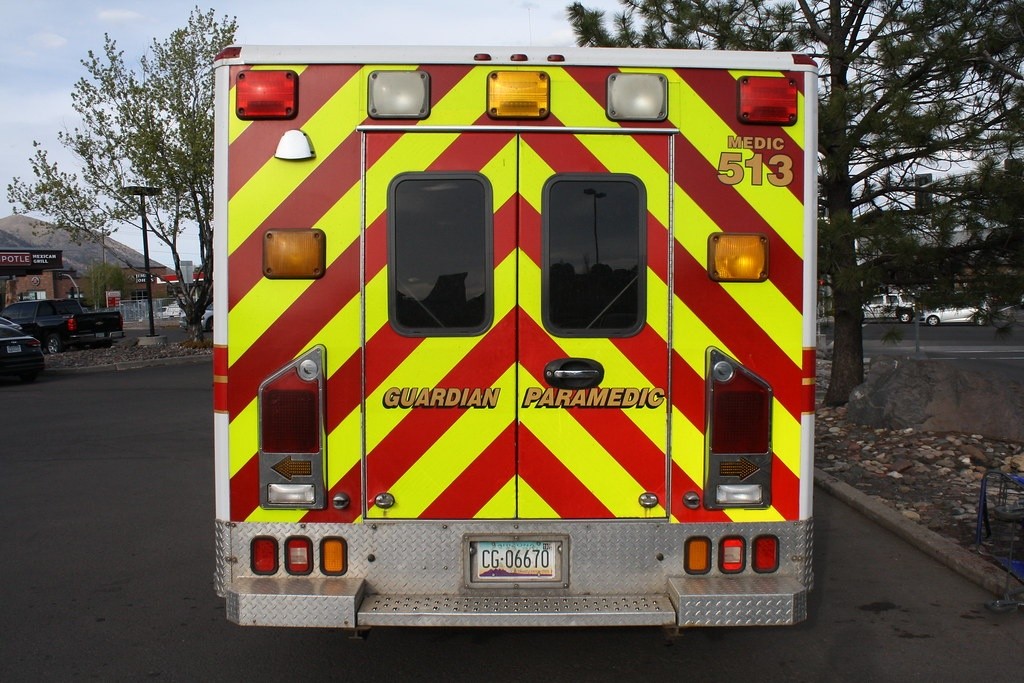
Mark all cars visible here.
[180,279,213,331]
[0,318,44,382]
[919,291,990,327]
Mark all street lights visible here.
[585,188,607,262]
[118,186,166,347]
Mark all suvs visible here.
[862,293,922,324]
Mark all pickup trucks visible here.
[0,298,123,354]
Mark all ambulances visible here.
[209,44,823,630]
